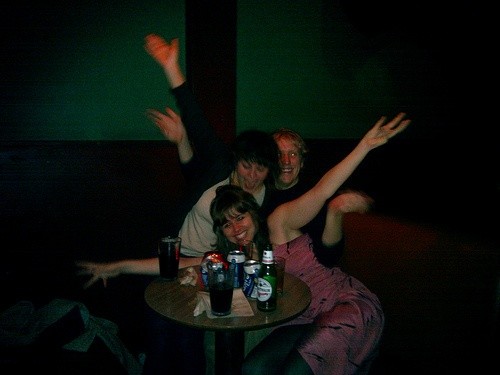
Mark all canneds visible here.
[200,256,223,290]
[242,260,261,300]
[227,250,245,288]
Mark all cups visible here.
[239,240,253,260]
[158,236,181,281]
[274,256,286,292]
[207,263,236,316]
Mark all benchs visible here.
[341,212,500,375]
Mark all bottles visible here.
[257,244,277,311]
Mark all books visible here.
[198,288,254,319]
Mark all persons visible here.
[141,33,279,375]
[74,112,411,375]
[147,108,375,375]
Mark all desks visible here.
[143,265,313,375]
